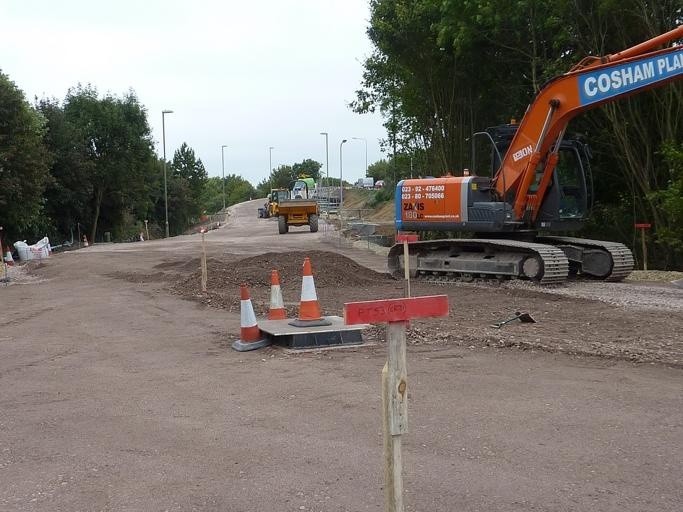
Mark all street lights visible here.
[338,139,348,206]
[319,132,328,187]
[221,145,227,208]
[352,136,367,178]
[268,146,274,195]
[160,109,176,237]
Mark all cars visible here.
[373,180,385,188]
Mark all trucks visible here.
[353,176,373,191]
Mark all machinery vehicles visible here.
[383,23,682,288]
[257,187,292,219]
[275,180,321,236]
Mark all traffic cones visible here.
[290,257,333,327]
[263,268,289,322]
[232,283,270,355]
[4,245,14,266]
[82,234,88,247]
